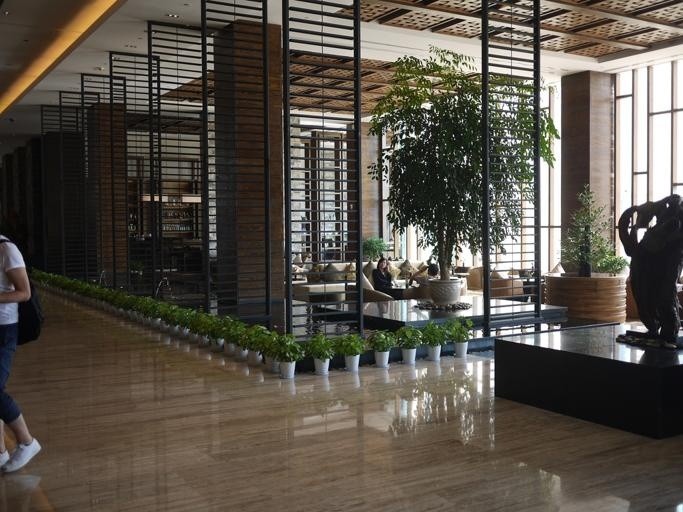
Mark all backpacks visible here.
[0,239,45,346]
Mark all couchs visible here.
[290,252,523,303]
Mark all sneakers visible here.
[1,436,44,475]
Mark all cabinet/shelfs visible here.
[162,193,193,234]
[126,178,142,236]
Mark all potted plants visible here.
[365,44,562,304]
[275,312,475,379]
[26,267,276,379]
[542,181,630,328]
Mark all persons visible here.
[373,258,393,297]
[412,263,439,288]
[0,234,43,473]
